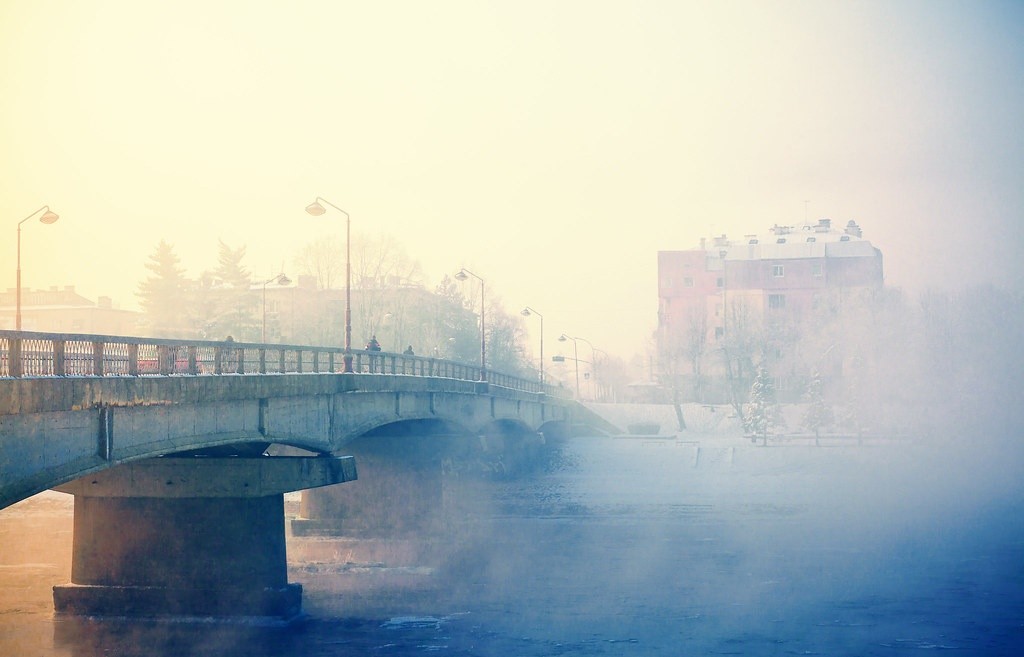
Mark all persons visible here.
[365,336,381,373]
[403,345,414,375]
[558,381,563,390]
[224,336,236,372]
